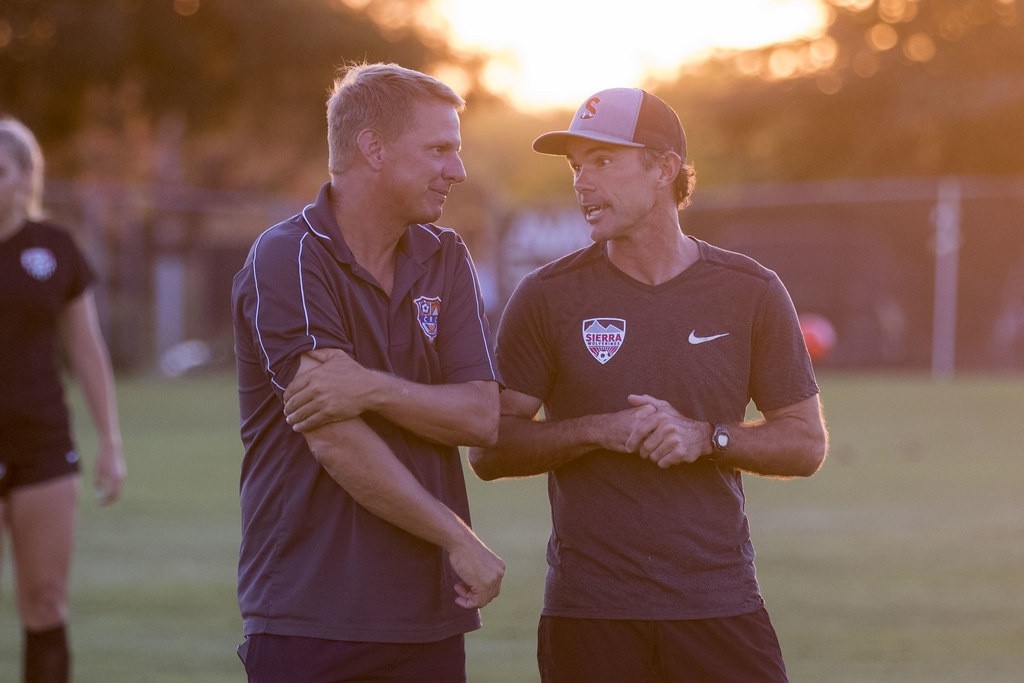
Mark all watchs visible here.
[706,421,731,463]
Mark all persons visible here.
[0,115,127,682]
[469,88,827,682]
[230,64,506,683]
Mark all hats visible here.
[532,88,687,166]
